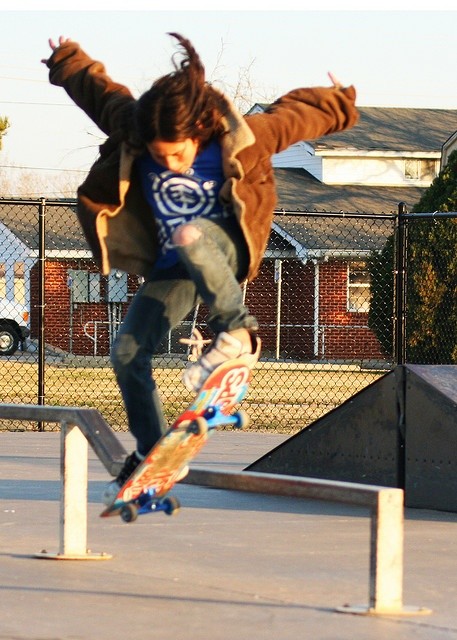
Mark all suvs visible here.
[0,297,30,356]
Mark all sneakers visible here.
[102,450,189,508]
[184,331,262,391]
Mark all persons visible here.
[38,32,361,508]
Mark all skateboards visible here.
[100,358,252,522]
